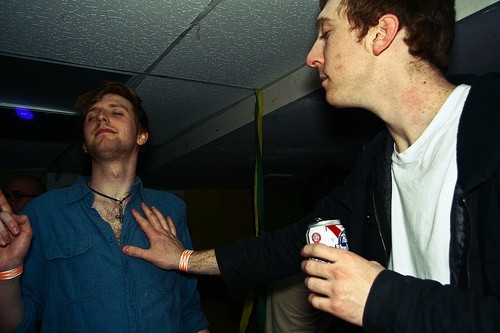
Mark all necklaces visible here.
[87,182,131,222]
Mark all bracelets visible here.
[0,265,24,281]
[179,249,195,273]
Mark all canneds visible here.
[306,217,350,266]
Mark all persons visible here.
[3,174,39,214]
[265,269,334,333]
[122,0,500,333]
[0,80,210,333]
